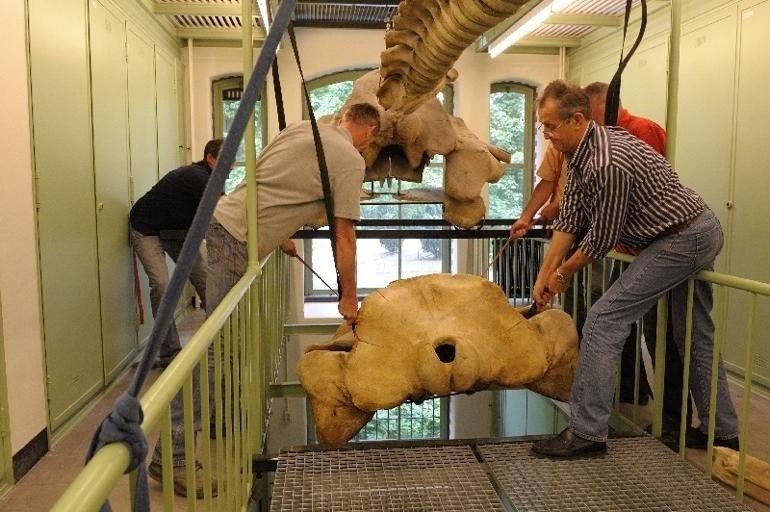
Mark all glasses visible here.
[534,116,571,138]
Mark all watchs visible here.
[553,269,570,286]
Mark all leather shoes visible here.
[684,426,741,453]
[530,426,608,461]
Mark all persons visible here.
[530,79,742,460]
[509,141,609,339]
[582,81,694,450]
[128,138,299,371]
[146,102,382,500]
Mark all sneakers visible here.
[147,457,228,499]
